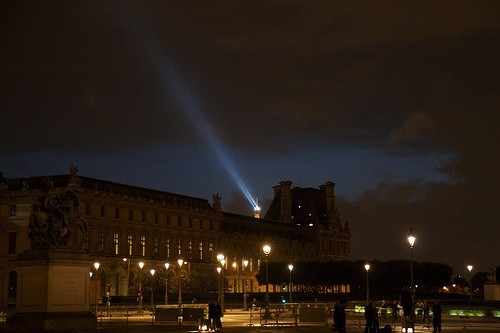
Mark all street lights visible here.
[287,263,294,303]
[176,258,184,316]
[93,261,100,316]
[407,236,417,307]
[466,265,474,300]
[242,259,250,311]
[216,267,223,316]
[232,261,238,293]
[363,262,371,305]
[164,262,170,305]
[262,243,273,320]
[149,268,156,315]
[216,254,227,313]
[137,261,145,313]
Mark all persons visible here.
[364,302,379,333]
[209,300,223,330]
[432,299,442,333]
[416,300,424,315]
[381,300,387,315]
[392,300,398,319]
[423,300,431,324]
[333,299,346,333]
